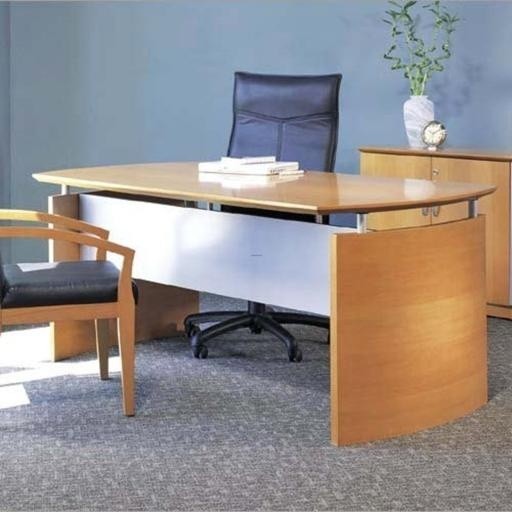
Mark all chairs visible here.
[1,209,138,417]
[184,71,343,361]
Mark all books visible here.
[200,172,304,188]
[197,156,305,176]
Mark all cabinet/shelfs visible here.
[358,146,511,320]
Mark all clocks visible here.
[421,120,447,146]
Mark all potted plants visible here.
[383,1,468,148]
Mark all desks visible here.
[31,162,499,447]
